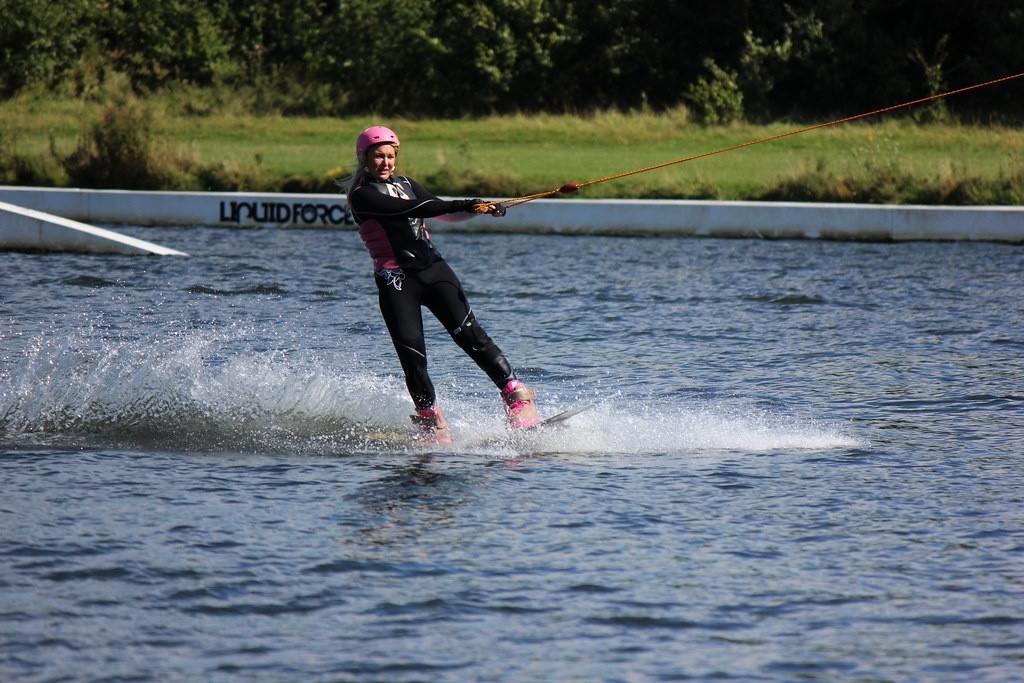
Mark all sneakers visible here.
[502,378,539,430]
[417,406,453,444]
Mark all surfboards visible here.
[360,390,622,448]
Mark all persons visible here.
[349,125,536,442]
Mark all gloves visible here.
[455,198,507,217]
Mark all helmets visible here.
[356,126,400,171]
